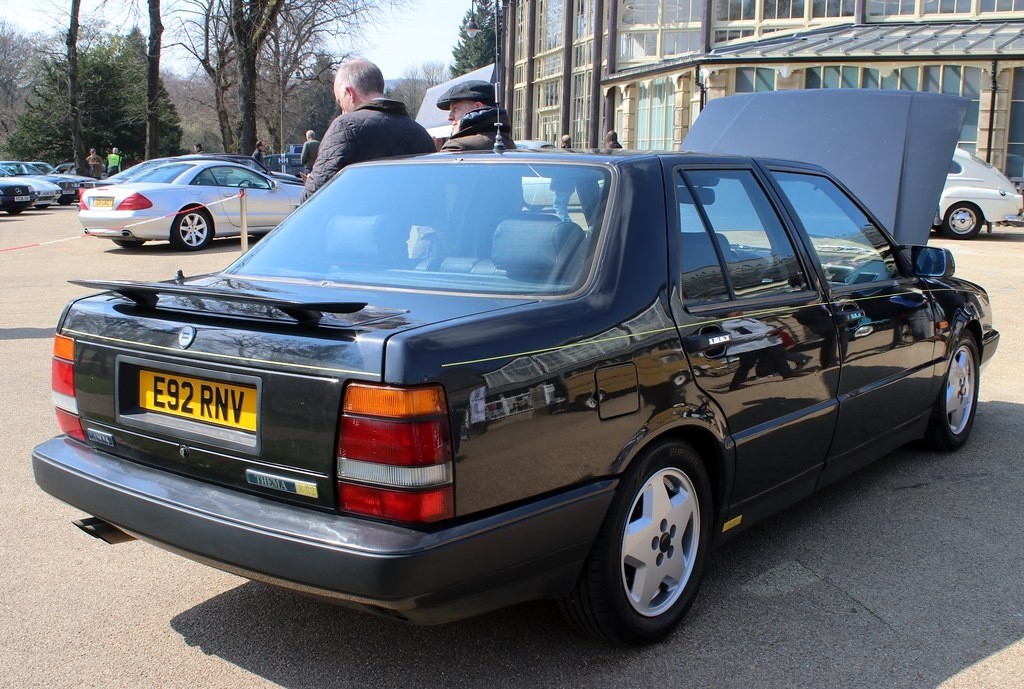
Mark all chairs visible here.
[681,228,738,299]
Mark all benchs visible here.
[323,215,585,287]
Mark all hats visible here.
[113,148,119,154]
[436,80,494,111]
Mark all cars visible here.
[0,143,310,217]
[31,81,1004,650]
[78,160,305,253]
[512,139,607,212]
[931,149,1024,242]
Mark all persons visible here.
[301,130,320,176]
[436,80,524,259]
[722,311,818,393]
[252,140,265,171]
[194,143,204,153]
[105,148,124,177]
[788,276,840,368]
[86,149,102,180]
[891,280,933,340]
[550,135,575,221]
[605,131,623,149]
[297,59,438,269]
